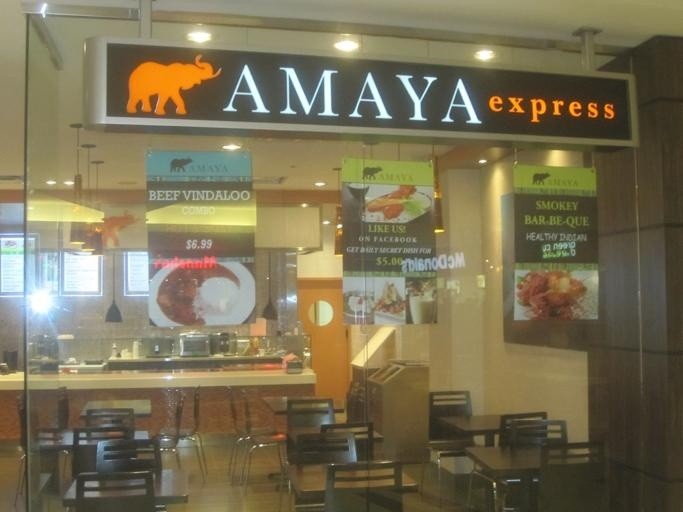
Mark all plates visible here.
[148,260,255,327]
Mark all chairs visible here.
[56,387,87,432]
[538,442,611,511]
[291,432,359,512]
[226,387,279,487]
[325,461,403,512]
[238,390,289,497]
[145,391,180,471]
[510,420,568,447]
[85,408,135,433]
[468,412,550,512]
[16,394,78,506]
[95,440,162,473]
[281,398,337,494]
[158,384,208,482]
[319,423,374,462]
[420,391,481,507]
[76,472,156,512]
[72,428,132,482]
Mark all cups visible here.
[409,295,437,324]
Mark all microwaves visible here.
[179,334,209,357]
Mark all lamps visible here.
[69,123,85,242]
[333,166,342,257]
[431,143,443,232]
[82,143,96,251]
[263,253,277,321]
[91,157,102,255]
[105,254,120,322]
